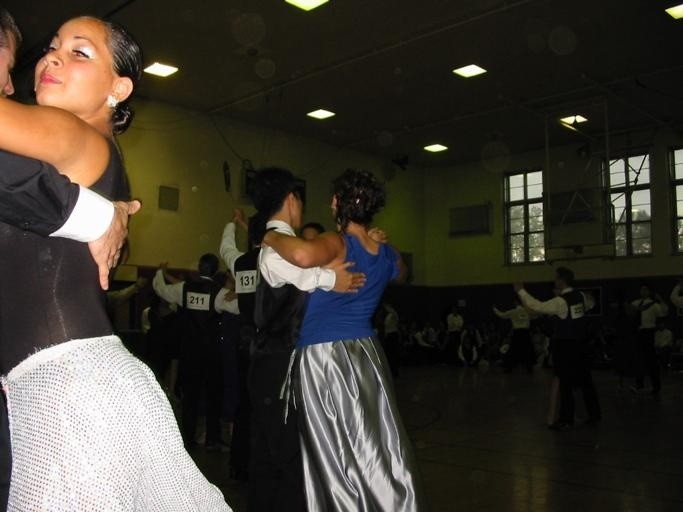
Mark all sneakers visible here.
[549,418,601,430]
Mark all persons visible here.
[263,170,421,511]
[0,15,236,511]
[104,210,326,481]
[0,5,140,511]
[370,266,681,434]
[247,167,386,510]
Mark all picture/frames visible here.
[574,286,603,318]
